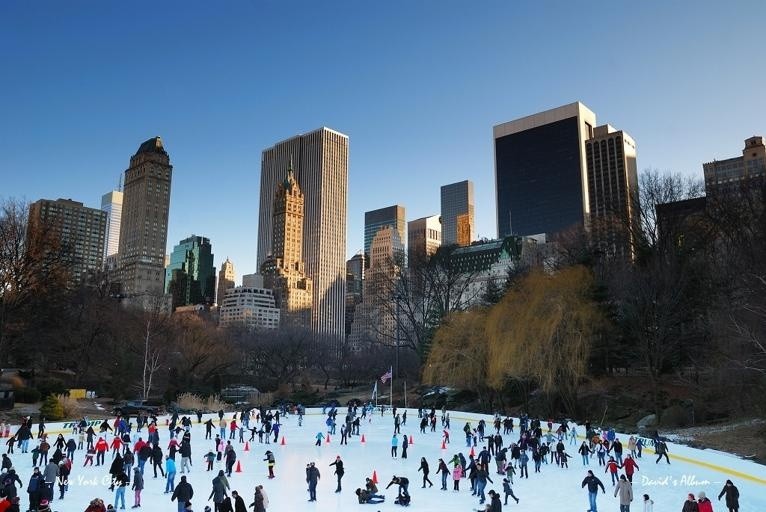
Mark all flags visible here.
[381,368,391,383]
[371,383,377,399]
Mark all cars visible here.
[421,382,462,396]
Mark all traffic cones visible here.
[234,460,242,474]
[441,440,446,448]
[326,433,331,443]
[370,470,378,483]
[468,446,477,458]
[408,433,414,443]
[360,433,365,442]
[280,435,286,446]
[244,440,249,450]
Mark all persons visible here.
[1,399,741,511]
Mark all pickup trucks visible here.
[113,397,165,416]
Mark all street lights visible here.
[390,291,407,385]
[108,290,129,355]
